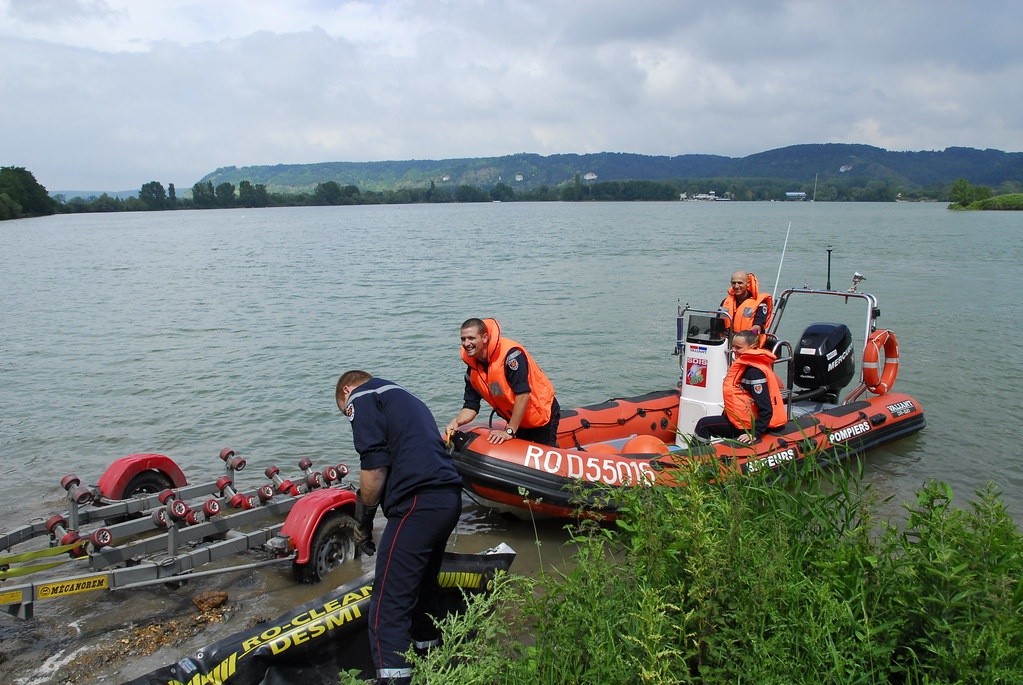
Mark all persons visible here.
[689,330,787,448]
[335,369,465,685]
[445,318,560,446]
[716,271,773,350]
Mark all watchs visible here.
[504,427,516,438]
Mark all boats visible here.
[441,244,925,522]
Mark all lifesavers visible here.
[861,329,900,395]
